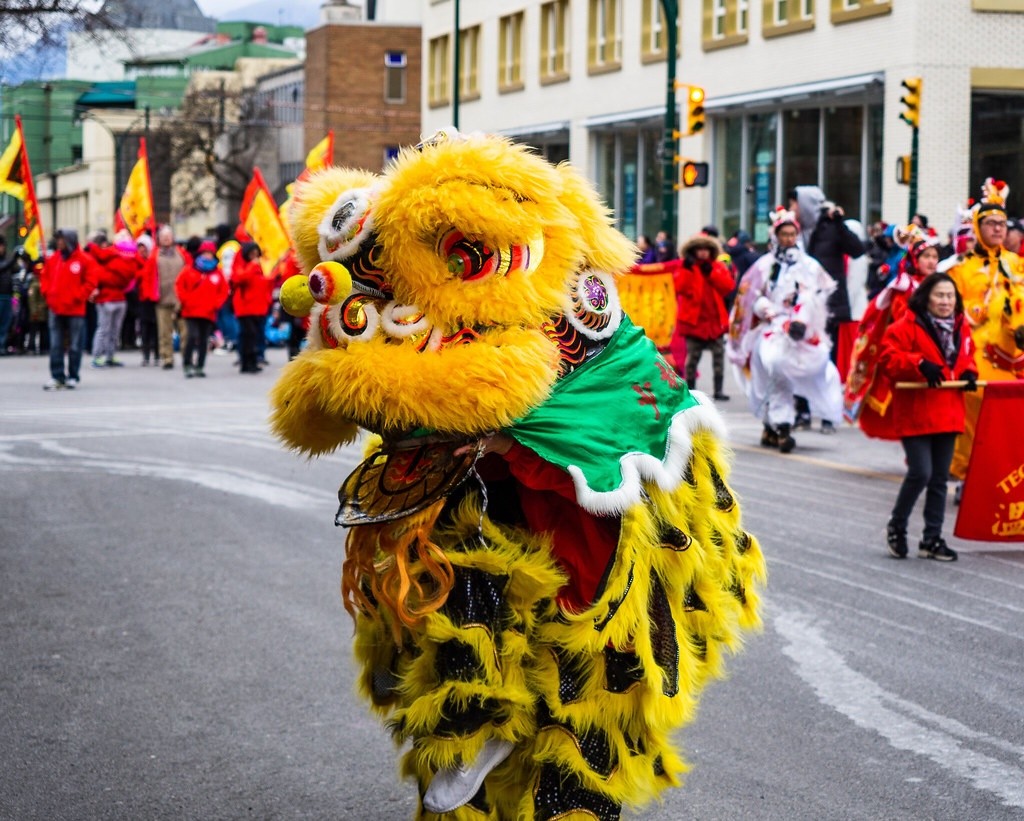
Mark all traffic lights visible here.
[687,88,707,136]
[896,157,911,183]
[682,163,711,188]
[900,77,921,126]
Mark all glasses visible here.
[981,219,1009,229]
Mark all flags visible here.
[119,156,151,242]
[236,177,293,277]
[0,119,30,201]
[306,135,327,175]
[21,176,41,261]
[613,268,678,348]
[953,383,1024,545]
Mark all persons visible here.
[636,224,736,400]
[176,233,200,263]
[40,233,99,390]
[174,240,231,377]
[282,254,312,362]
[0,235,49,356]
[82,224,185,370]
[231,243,274,375]
[727,174,1024,562]
[725,231,764,311]
[211,225,242,353]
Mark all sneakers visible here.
[918,538,956,561]
[885,516,909,558]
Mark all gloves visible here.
[701,261,713,275]
[918,359,946,389]
[959,369,978,392]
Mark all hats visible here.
[198,240,216,254]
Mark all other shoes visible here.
[106,360,123,366]
[141,359,150,366]
[44,379,61,391]
[713,393,729,400]
[241,365,263,373]
[66,378,77,389]
[91,360,106,368]
[196,369,206,379]
[183,366,195,378]
[760,429,780,446]
[779,435,794,454]
[154,358,159,366]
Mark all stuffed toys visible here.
[269,130,770,821]
